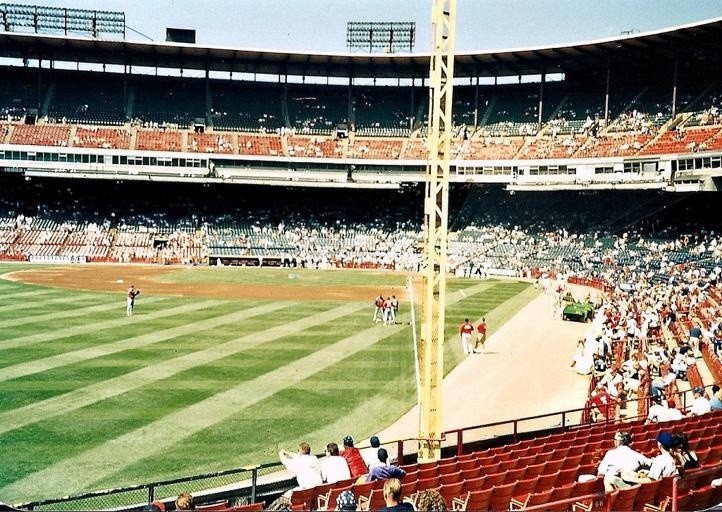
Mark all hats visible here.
[656,432,674,447]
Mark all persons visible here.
[459,318,475,354]
[450,105,722,159]
[475,317,487,349]
[597,429,698,494]
[127,286,136,315]
[446,206,563,294]
[1,106,426,178]
[373,295,399,326]
[553,217,722,424]
[1,197,423,272]
[279,435,448,512]
[175,493,193,511]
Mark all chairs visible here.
[3,61,722,161]
[1,166,722,282]
[182,420,722,512]
[590,279,722,420]
